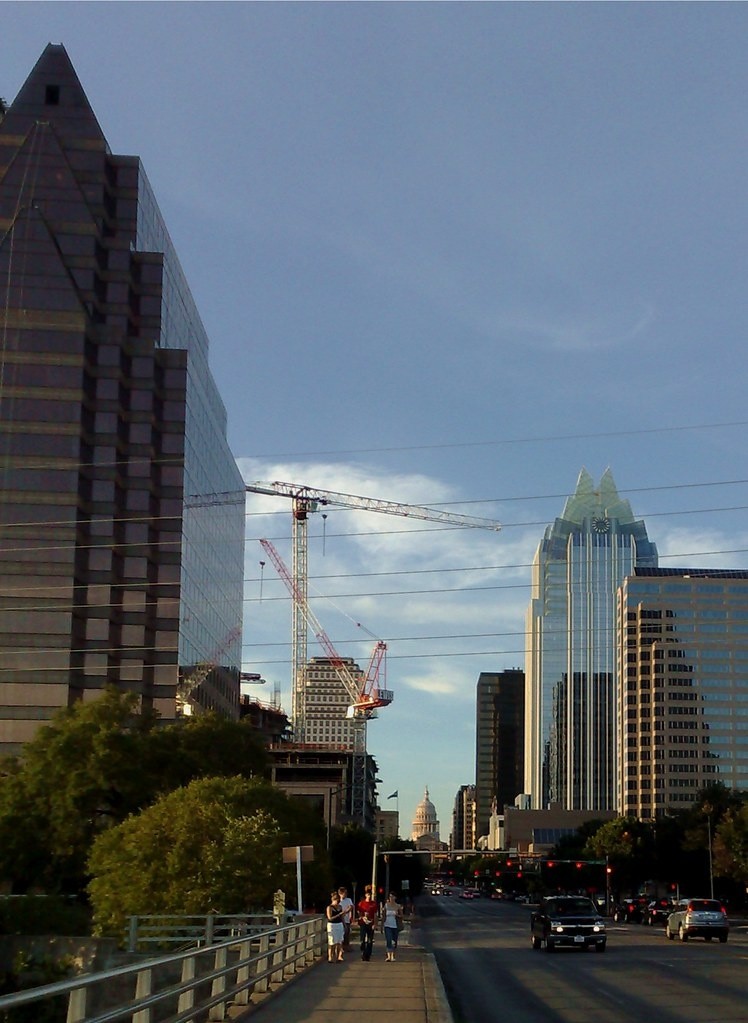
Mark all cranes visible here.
[244,480,502,748]
[240,672,265,685]
[258,540,393,831]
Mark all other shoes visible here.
[391,959,396,962]
[386,959,390,962]
[338,959,345,962]
[329,961,334,963]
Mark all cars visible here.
[613,899,644,923]
[640,900,674,926]
[443,888,452,896]
[458,891,463,897]
[431,888,440,896]
[473,890,480,898]
[436,883,445,888]
[462,891,473,900]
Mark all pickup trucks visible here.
[530,895,607,954]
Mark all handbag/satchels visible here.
[396,917,404,931]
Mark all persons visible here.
[336,887,354,953]
[357,890,378,961]
[381,892,403,962]
[326,892,348,963]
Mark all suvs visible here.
[666,899,729,943]
